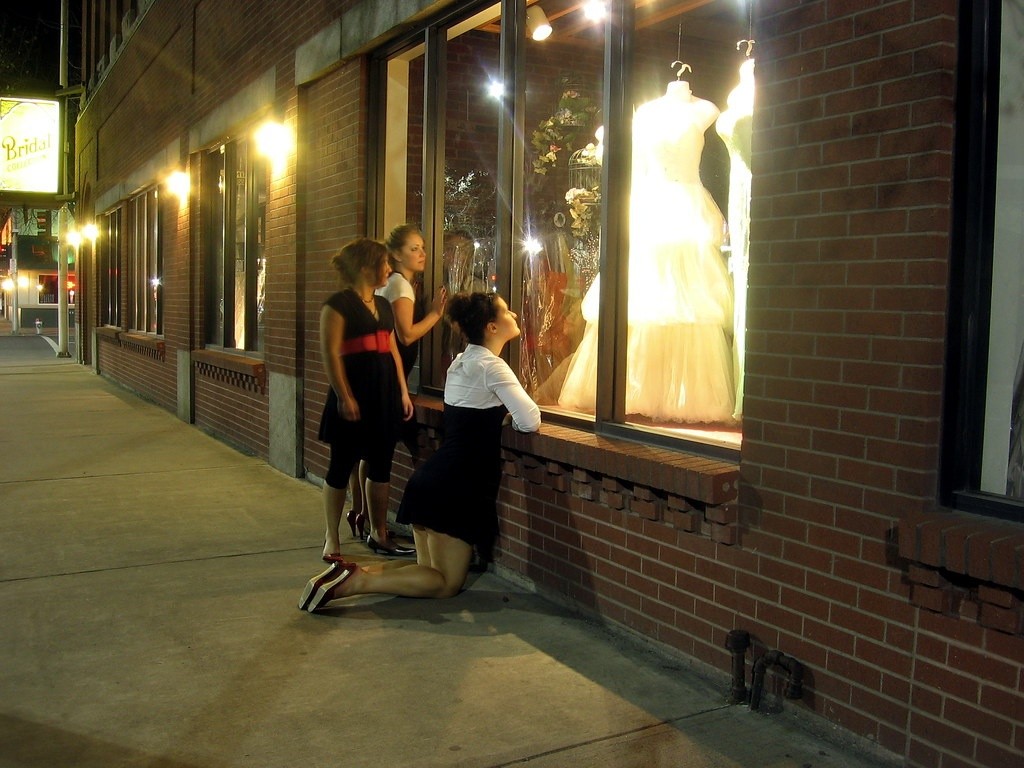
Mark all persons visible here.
[443,229,489,365]
[346,224,446,540]
[318,241,417,562]
[559,57,754,425]
[298,289,541,614]
[533,298,586,405]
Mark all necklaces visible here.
[361,296,376,316]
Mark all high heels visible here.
[322,539,344,556]
[346,510,359,536]
[298,556,357,613]
[355,513,396,539]
[367,535,416,555]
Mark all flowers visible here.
[530,89,602,237]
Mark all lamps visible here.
[525,6,552,40]
